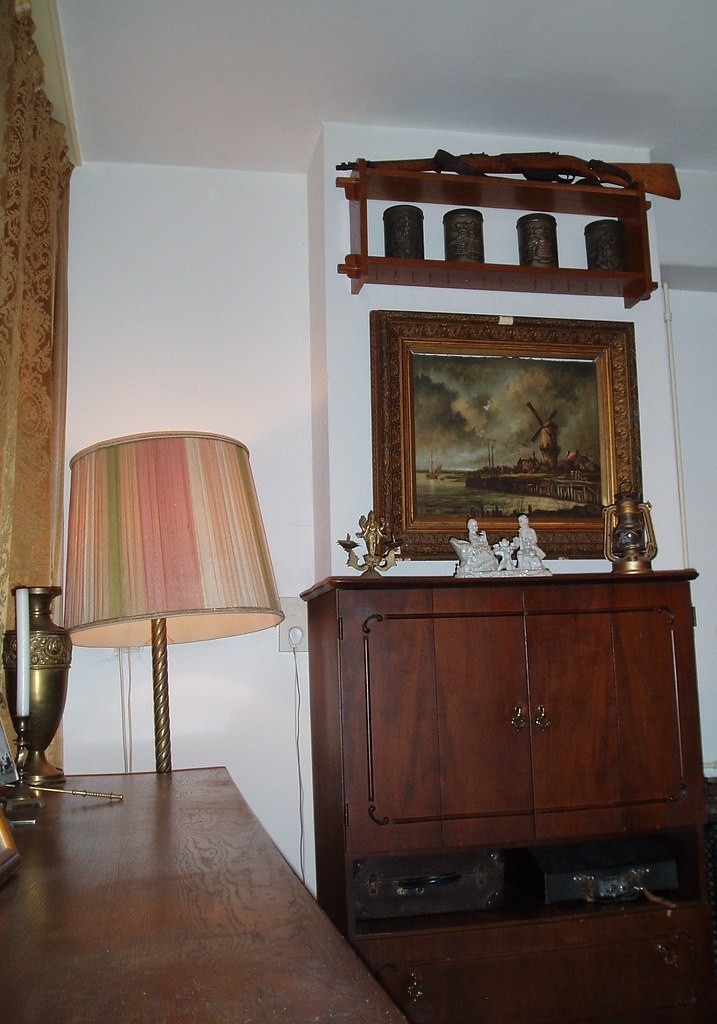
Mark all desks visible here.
[1,766,407,1023]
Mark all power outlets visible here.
[278,597,306,652]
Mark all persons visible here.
[517,515,539,570]
[468,520,499,570]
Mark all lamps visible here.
[62,431,284,773]
[601,491,657,575]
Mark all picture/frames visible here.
[369,310,645,561]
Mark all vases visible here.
[3,586,71,791]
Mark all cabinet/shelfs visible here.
[297,569,716,1024]
[335,158,658,310]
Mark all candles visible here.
[15,588,30,717]
